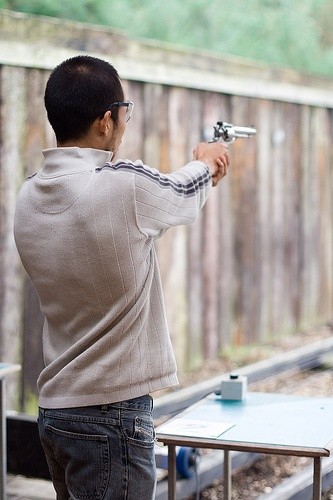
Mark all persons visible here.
[12,55,230,499]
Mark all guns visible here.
[209,120,260,146]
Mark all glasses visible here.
[99,100,134,123]
